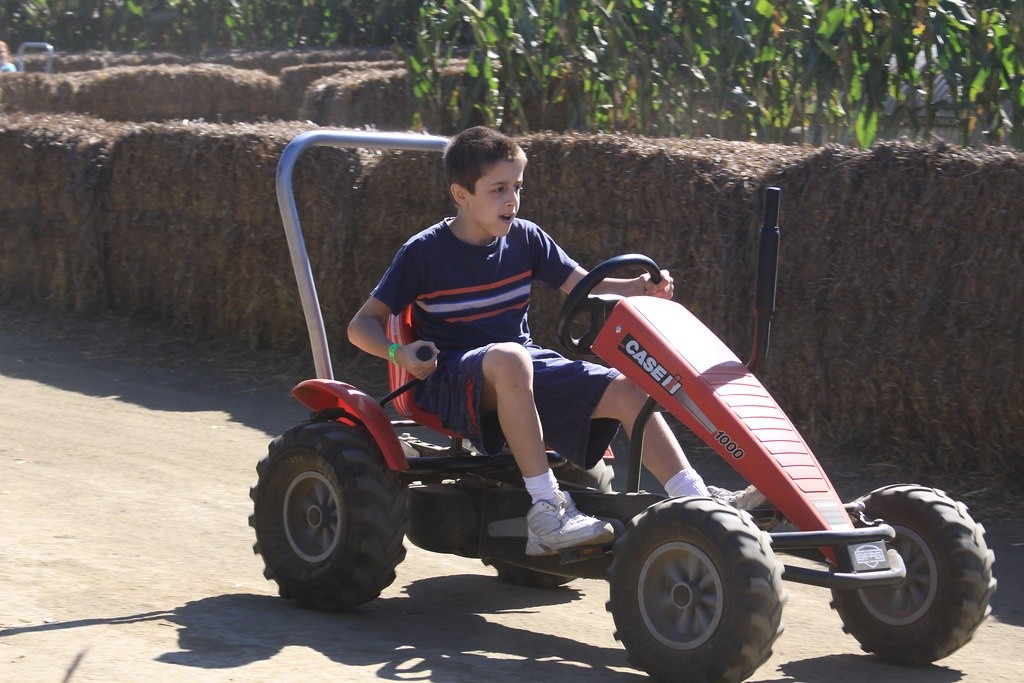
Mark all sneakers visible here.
[708,482,766,511]
[525,492,616,555]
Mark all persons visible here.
[348,126,767,557]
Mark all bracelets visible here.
[388,344,401,366]
